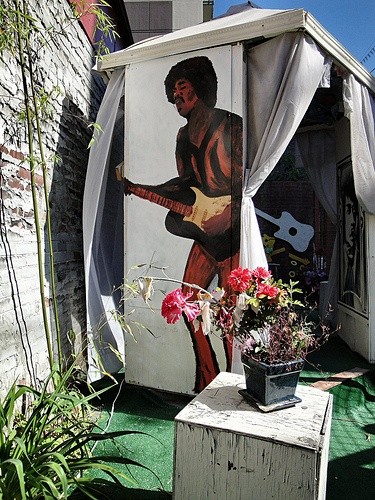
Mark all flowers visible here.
[134,266,343,372]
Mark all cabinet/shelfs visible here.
[173,372,333,500]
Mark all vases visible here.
[240,350,302,406]
